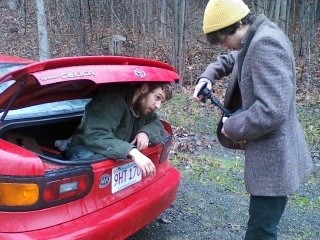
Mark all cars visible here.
[1,53,181,240]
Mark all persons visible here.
[193,0,313,240]
[64,83,172,179]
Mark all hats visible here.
[201,0,251,36]
